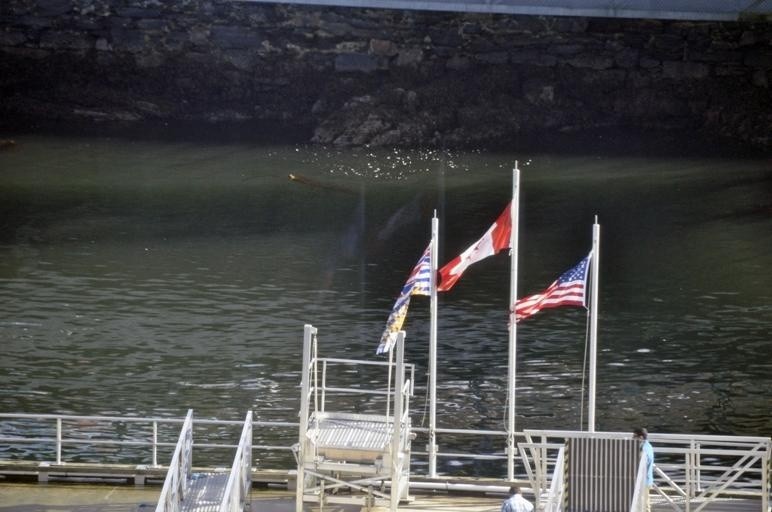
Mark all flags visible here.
[508,254,590,326]
[436,200,512,292]
[375,240,432,356]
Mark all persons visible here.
[632,427,654,512]
[501,484,534,512]
